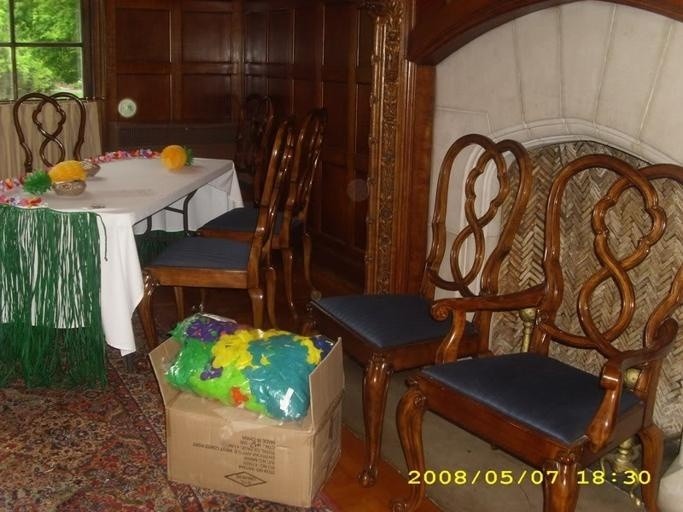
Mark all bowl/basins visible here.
[77,160,100,177]
[52,180,87,197]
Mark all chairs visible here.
[232,93,279,208]
[14,93,86,174]
[198,107,328,323]
[302,134,532,488]
[390,153,683,512]
[137,114,298,350]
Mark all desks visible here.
[0,154,243,373]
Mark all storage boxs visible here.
[146,334,345,510]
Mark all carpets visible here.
[1,306,445,512]
